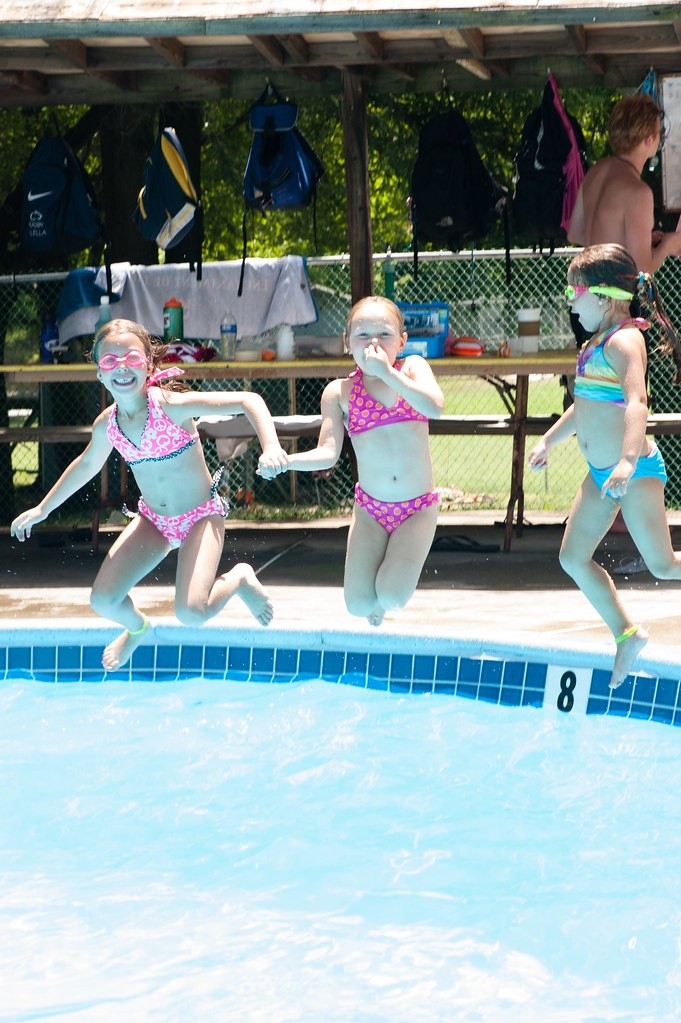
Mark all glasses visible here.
[98,351,148,372]
[565,285,593,300]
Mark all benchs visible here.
[0,412,680,444]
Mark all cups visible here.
[517,309,542,353]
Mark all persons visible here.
[11,319,288,671]
[568,96,680,348]
[259,295,445,627]
[529,242,681,689]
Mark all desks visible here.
[1,350,586,555]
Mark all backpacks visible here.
[0,113,100,266]
[512,73,590,240]
[223,83,325,209]
[411,85,506,244]
[131,105,202,253]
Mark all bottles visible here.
[94,304,112,363]
[163,297,183,345]
[40,313,59,364]
[220,310,237,361]
[276,323,295,362]
